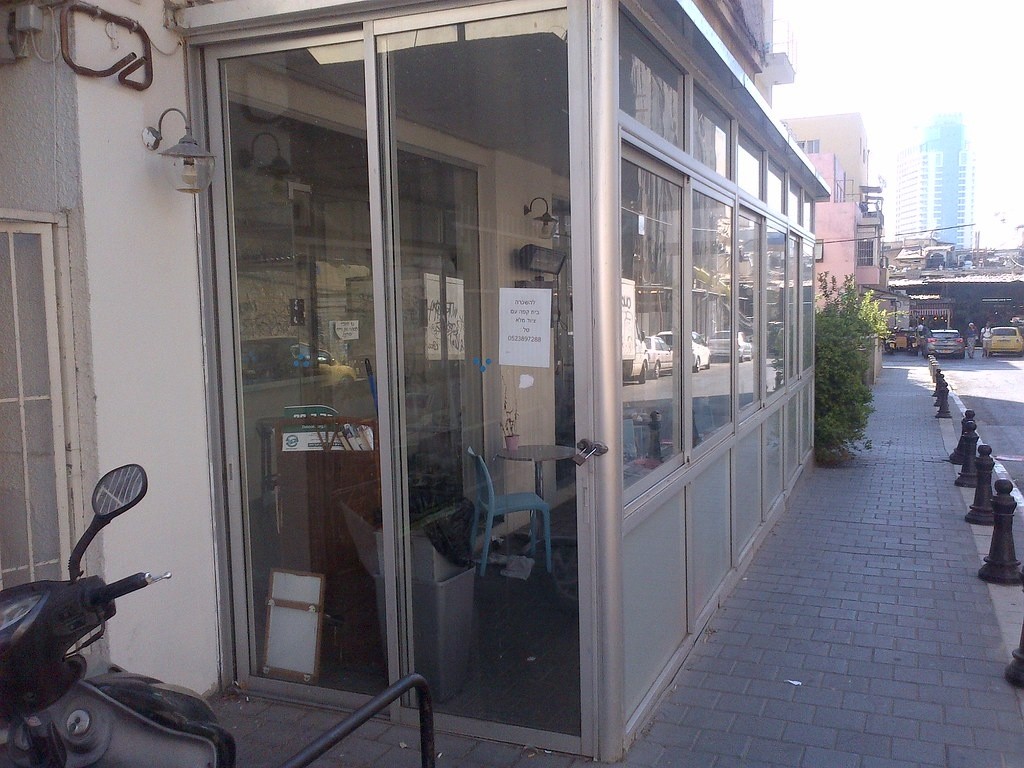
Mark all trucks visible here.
[621,278,648,385]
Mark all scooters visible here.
[0,464,239,768]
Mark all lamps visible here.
[518,245,568,277]
[235,129,293,181]
[522,197,558,241]
[142,108,218,193]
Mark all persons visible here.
[980,321,994,359]
[917,325,933,360]
[964,323,978,359]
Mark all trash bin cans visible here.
[376,561,476,706]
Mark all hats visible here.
[969,323,974,326]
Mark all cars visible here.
[317,350,356,386]
[922,330,965,359]
[708,330,752,363]
[644,336,673,379]
[988,326,1024,357]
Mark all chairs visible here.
[466,447,552,576]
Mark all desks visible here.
[495,445,574,564]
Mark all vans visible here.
[659,330,711,373]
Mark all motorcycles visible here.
[884,327,898,354]
[907,327,920,355]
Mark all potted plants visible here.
[499,376,520,453]
